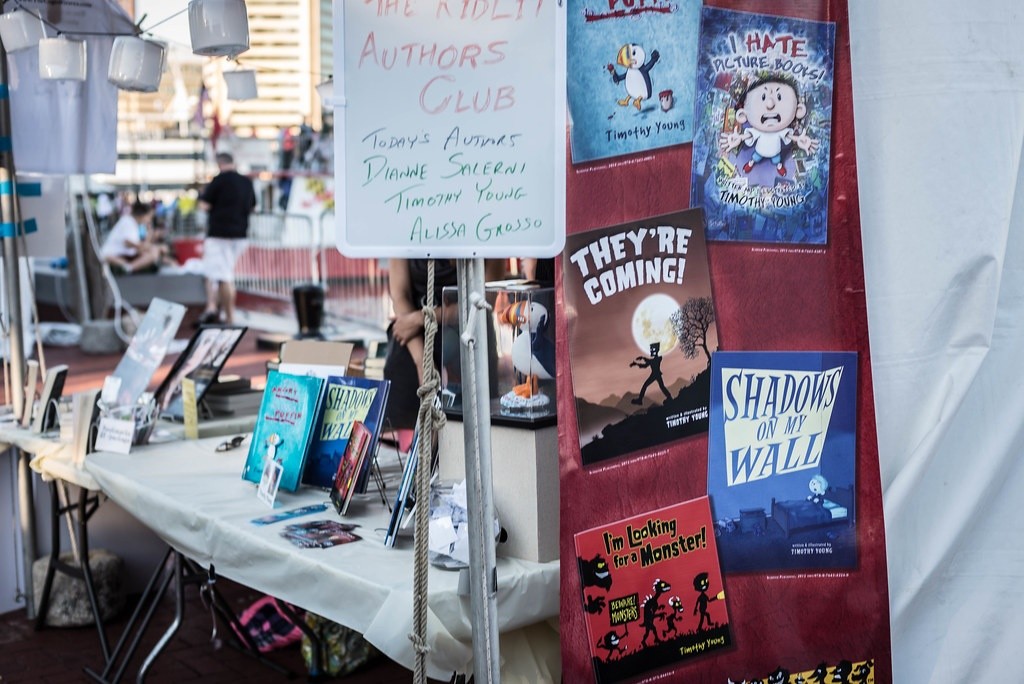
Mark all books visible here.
[242,363,392,517]
[74,389,102,455]
[21,359,38,426]
[384,388,456,548]
[33,365,68,432]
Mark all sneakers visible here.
[190,310,218,329]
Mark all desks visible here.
[0,386,564,684]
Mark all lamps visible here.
[0,0,257,101]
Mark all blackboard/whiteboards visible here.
[331,1,566,258]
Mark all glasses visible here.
[215,437,246,452]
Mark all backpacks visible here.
[298,609,379,681]
[228,594,305,655]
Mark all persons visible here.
[198,153,259,324]
[157,327,220,412]
[99,203,161,277]
[384,259,461,452]
[495,257,557,388]
